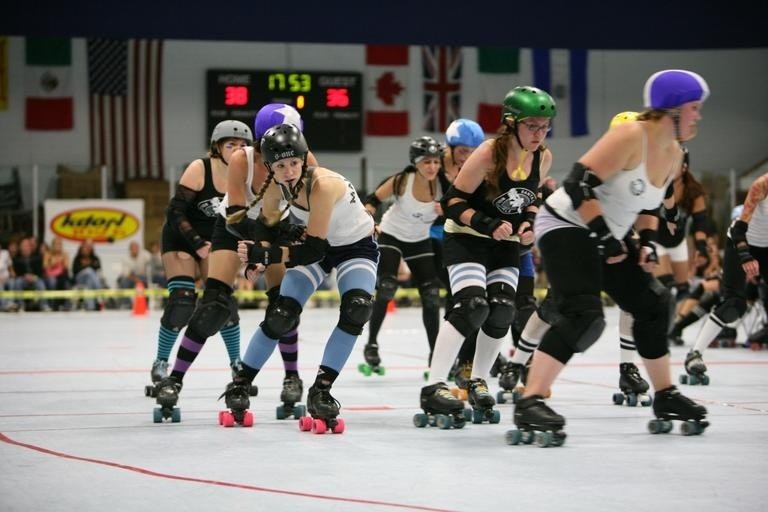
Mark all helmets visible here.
[408,136,442,164]
[608,112,642,129]
[255,103,304,142]
[210,120,254,147]
[642,69,711,113]
[445,119,485,149]
[500,86,558,125]
[260,124,309,165]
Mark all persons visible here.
[409,81,558,428]
[143,117,271,400]
[503,69,714,449]
[218,126,379,435]
[152,101,313,425]
[0,236,268,312]
[366,111,768,400]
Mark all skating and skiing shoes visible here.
[613,363,652,407]
[647,385,710,437]
[679,351,710,387]
[357,342,385,378]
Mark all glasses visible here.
[520,120,553,134]
